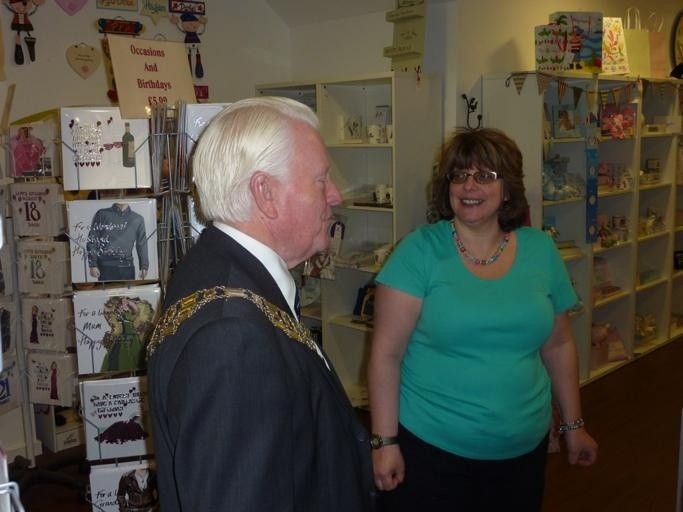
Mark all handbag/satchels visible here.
[623,6,667,80]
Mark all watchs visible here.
[370,433,399,450]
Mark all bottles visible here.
[121,121,134,169]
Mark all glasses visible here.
[444,169,503,185]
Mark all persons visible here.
[146,97,375,512]
[367,126,599,512]
[88,203,149,281]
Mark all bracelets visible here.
[557,419,586,436]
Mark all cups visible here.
[366,123,382,145]
[376,183,392,207]
[372,248,388,267]
[384,124,392,143]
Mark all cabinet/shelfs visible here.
[477,72,683,392]
[253,70,444,417]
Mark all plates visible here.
[290,266,319,307]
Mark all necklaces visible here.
[452,220,511,266]
